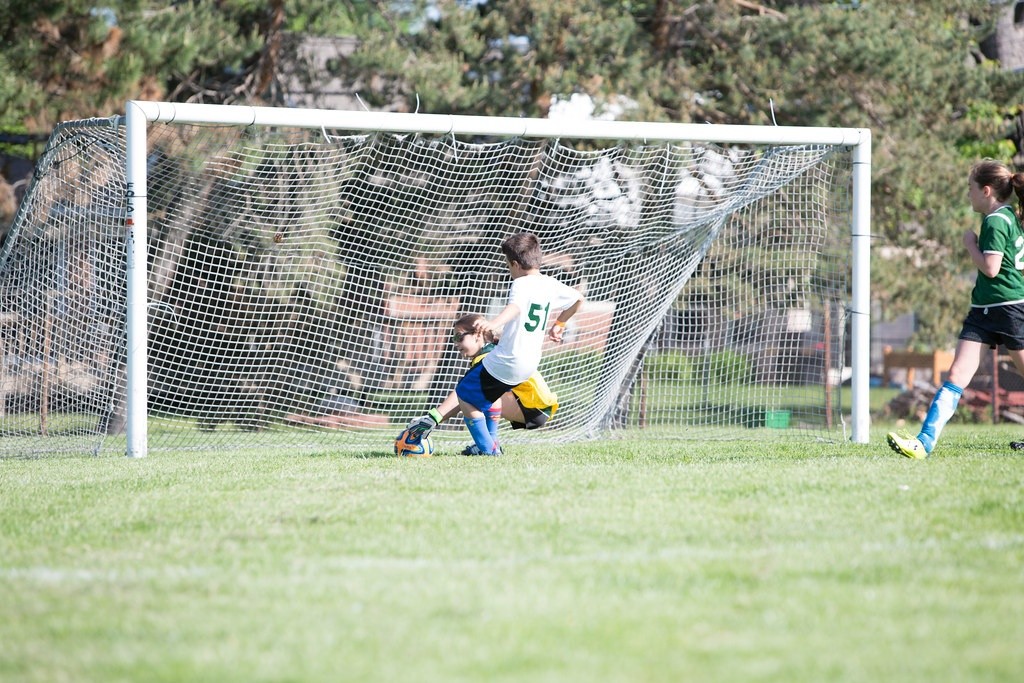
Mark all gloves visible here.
[408,408,443,439]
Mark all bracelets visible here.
[555,320,566,327]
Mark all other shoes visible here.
[461,444,501,457]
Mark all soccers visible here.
[393,428,435,460]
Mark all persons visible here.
[456,232,584,456]
[886,160,1024,459]
[406,313,559,456]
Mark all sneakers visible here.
[1010,441,1024,451]
[886,429,927,460]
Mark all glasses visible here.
[452,332,474,342]
